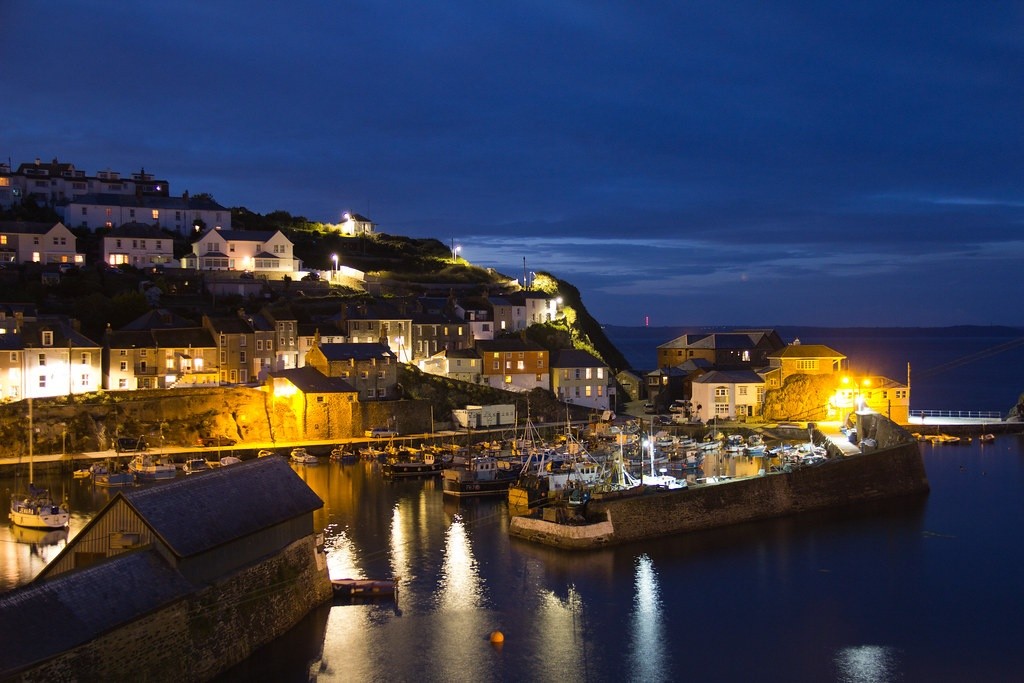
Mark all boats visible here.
[912,429,997,447]
[73,440,385,488]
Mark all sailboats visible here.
[7,399,72,529]
[378,398,830,508]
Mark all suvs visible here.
[364,427,399,438]
[115,438,148,454]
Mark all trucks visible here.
[201,434,238,447]
